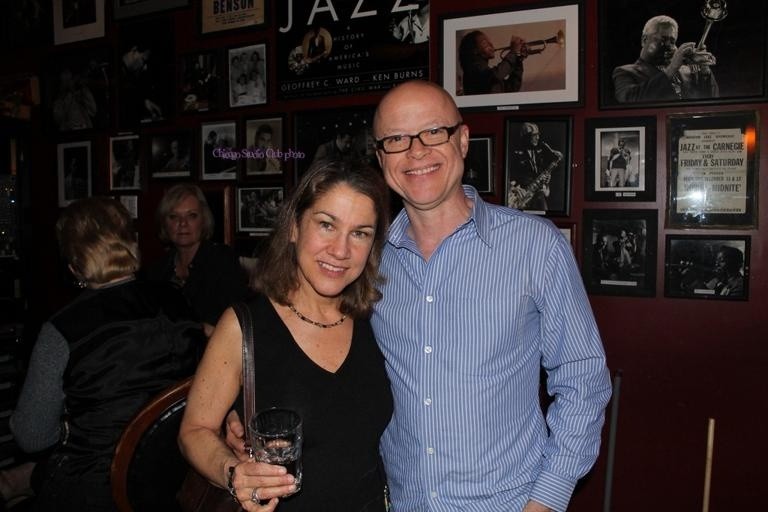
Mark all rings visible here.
[250,487,261,503]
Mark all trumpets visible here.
[491,29,566,59]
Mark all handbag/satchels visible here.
[172,302,258,511]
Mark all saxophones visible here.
[508,141,563,209]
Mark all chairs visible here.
[114,372,231,512]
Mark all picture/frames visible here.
[57,141,92,207]
[499,115,572,215]
[110,134,145,193]
[235,184,284,234]
[245,115,284,178]
[576,206,658,297]
[594,0,768,112]
[202,185,231,246]
[227,42,270,110]
[664,235,752,298]
[579,117,657,201]
[201,121,239,182]
[436,0,587,114]
[289,101,391,197]
[200,3,267,35]
[34,1,222,133]
[459,133,492,200]
[144,132,194,179]
[662,110,761,233]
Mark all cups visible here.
[250,406,303,498]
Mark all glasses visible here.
[370,120,465,155]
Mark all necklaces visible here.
[171,259,196,285]
[284,296,350,330]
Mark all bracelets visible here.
[224,458,248,505]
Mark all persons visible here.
[150,182,251,327]
[8,197,213,511]
[361,82,615,512]
[161,139,187,171]
[605,136,632,190]
[455,28,527,94]
[511,119,563,211]
[612,14,720,103]
[241,191,283,228]
[113,139,138,186]
[706,247,744,295]
[586,230,609,269]
[247,125,280,171]
[59,39,220,132]
[232,51,263,102]
[173,157,398,512]
[65,150,86,198]
[315,128,378,176]
[612,229,637,268]
[204,130,234,172]
